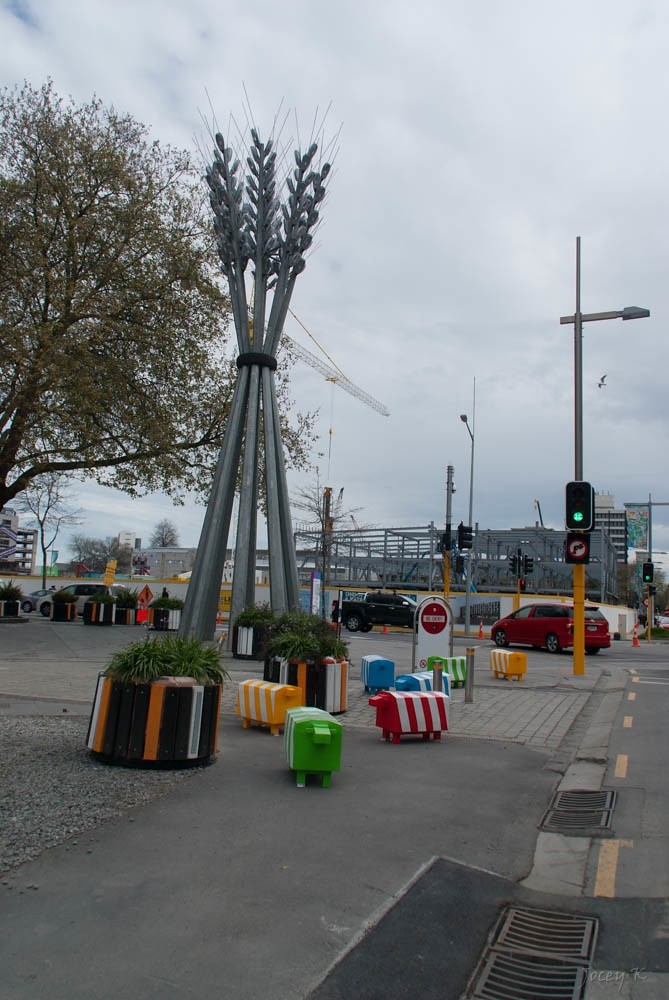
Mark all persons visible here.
[162,588,168,598]
[638,612,647,628]
[51,586,56,590]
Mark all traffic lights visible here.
[458,520,473,552]
[509,554,517,574]
[522,554,534,576]
[565,480,592,530]
[520,579,526,590]
[643,563,653,582]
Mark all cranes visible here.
[180,272,393,417]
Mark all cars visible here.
[490,600,612,655]
[654,615,669,630]
[19,589,57,612]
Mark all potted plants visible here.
[232,601,351,713]
[148,597,184,630]
[86,632,231,768]
[0,578,24,617]
[52,590,79,621]
[83,586,138,626]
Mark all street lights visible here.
[461,412,477,633]
[560,233,651,685]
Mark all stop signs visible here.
[420,603,449,635]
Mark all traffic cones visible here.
[217,608,223,624]
[382,624,389,635]
[332,622,337,632]
[632,625,642,647]
[477,621,485,640]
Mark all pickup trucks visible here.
[329,587,423,634]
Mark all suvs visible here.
[37,583,126,617]
